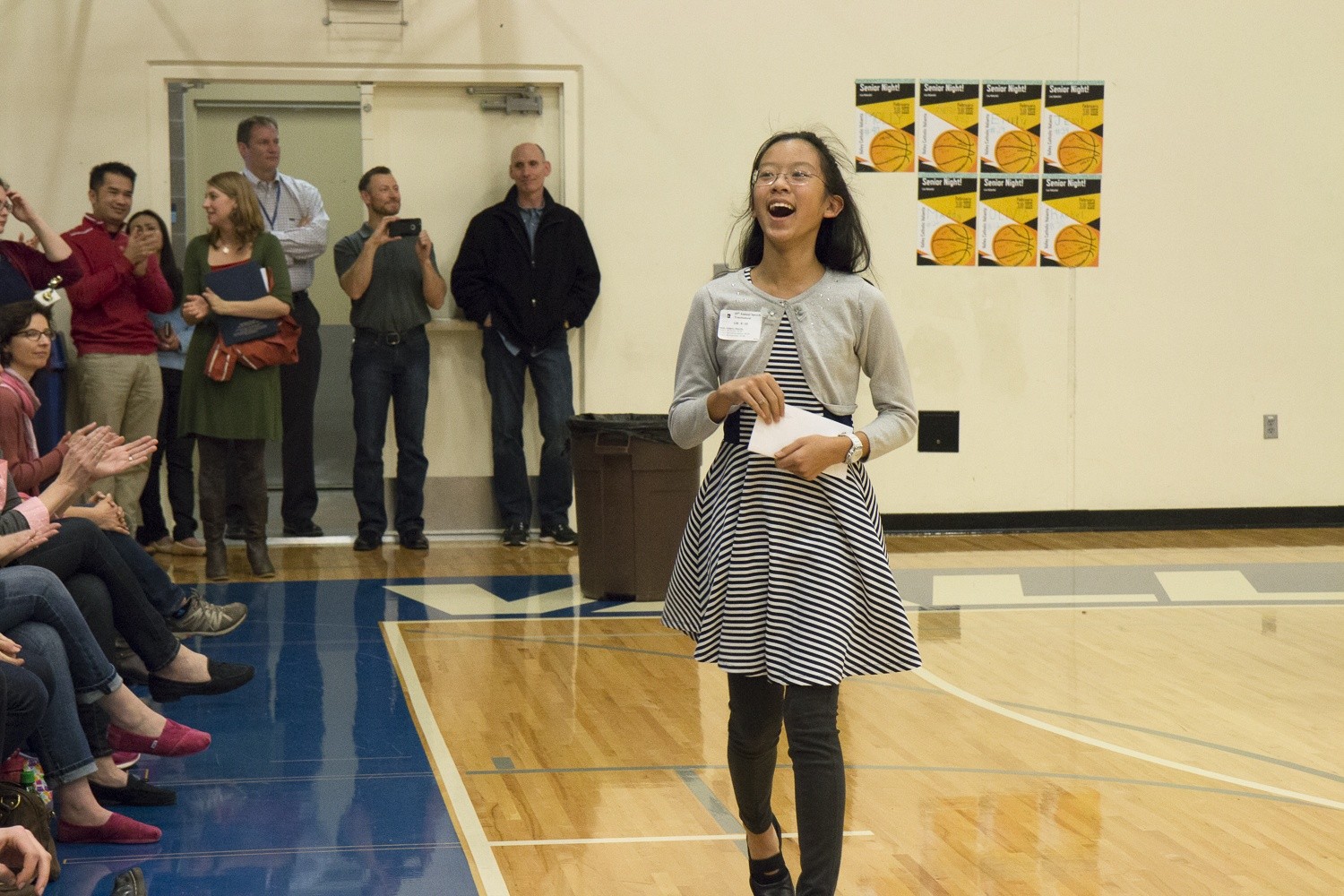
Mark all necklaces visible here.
[222,246,230,253]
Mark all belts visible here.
[355,327,425,346]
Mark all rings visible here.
[129,455,133,461]
[30,539,34,542]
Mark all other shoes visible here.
[400,528,429,549]
[87,772,176,806]
[283,516,324,537]
[746,811,797,896]
[107,709,211,756]
[115,660,150,685]
[143,538,175,553]
[171,542,206,556]
[111,747,141,768]
[353,531,381,551]
[225,523,247,540]
[56,808,162,844]
[108,868,145,896]
[147,640,255,702]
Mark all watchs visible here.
[837,431,864,466]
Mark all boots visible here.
[199,501,230,581]
[238,496,276,577]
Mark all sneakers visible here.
[539,521,578,545]
[502,521,531,545]
[162,586,249,641]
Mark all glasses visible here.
[12,327,57,342]
[0,200,12,213]
[751,170,832,193]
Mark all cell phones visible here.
[4,198,13,213]
[389,218,421,236]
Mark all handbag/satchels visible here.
[3,750,55,813]
[0,782,61,882]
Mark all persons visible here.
[123,205,210,557]
[176,172,294,580]
[0,179,254,896]
[450,142,601,547]
[333,167,448,552]
[660,131,923,896]
[59,161,173,542]
[226,116,332,540]
